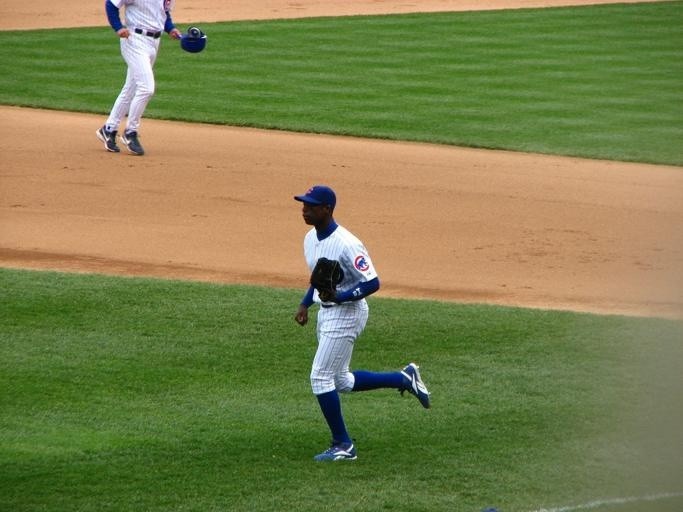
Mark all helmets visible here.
[178,27,206,52]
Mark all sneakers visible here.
[96,125,120,152]
[314,442,357,460]
[401,362,430,409]
[121,129,144,154]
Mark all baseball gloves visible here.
[310,257,344,302]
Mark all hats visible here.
[294,186,336,208]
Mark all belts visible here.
[135,28,161,39]
[321,304,332,308]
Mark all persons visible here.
[294,186,429,461]
[95,0,181,155]
